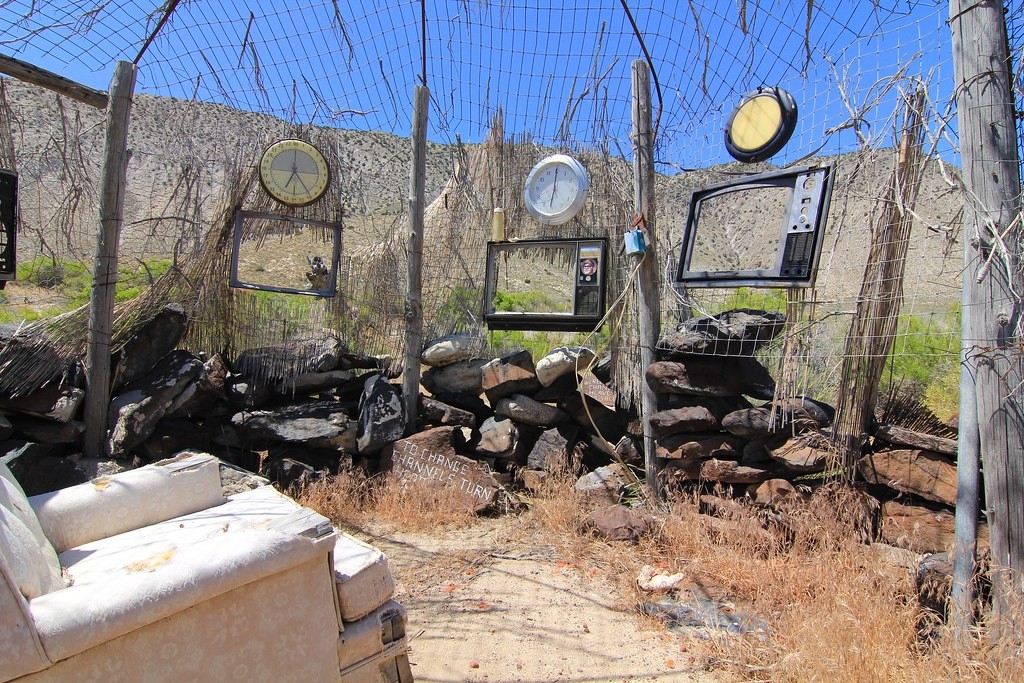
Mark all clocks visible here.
[258,138,331,206]
[523,154,588,226]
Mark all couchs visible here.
[0,449,413,682]
